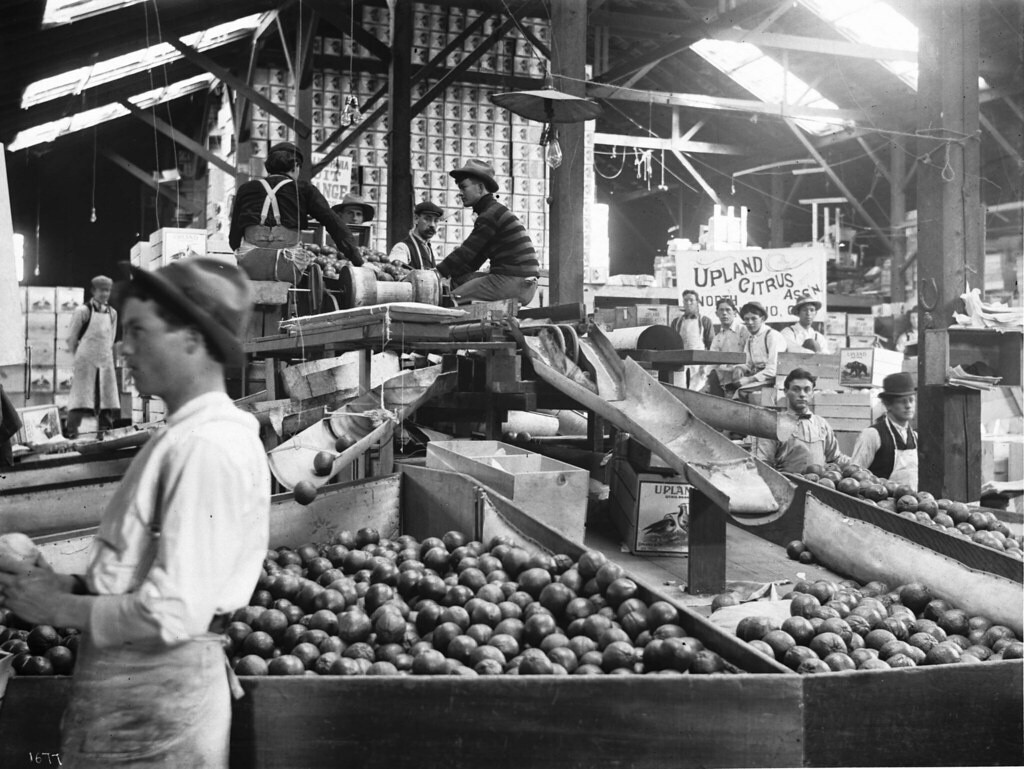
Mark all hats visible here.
[878,371,919,400]
[271,141,304,163]
[413,201,443,218]
[449,159,499,192]
[739,302,769,325]
[330,195,377,221]
[92,276,113,291]
[119,260,251,367]
[790,296,822,316]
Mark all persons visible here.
[781,293,828,354]
[435,158,539,305]
[229,142,364,285]
[389,202,444,270]
[896,306,918,357]
[330,193,375,224]
[851,372,918,492]
[670,290,788,402]
[0,255,273,769]
[64,276,120,431]
[753,368,841,473]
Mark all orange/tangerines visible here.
[301,241,412,282]
[714,453,1023,672]
[0,533,82,677]
[224,436,728,679]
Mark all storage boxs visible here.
[608,425,694,557]
[615,302,919,430]
[425,440,590,545]
[0,286,98,446]
[130,3,610,429]
